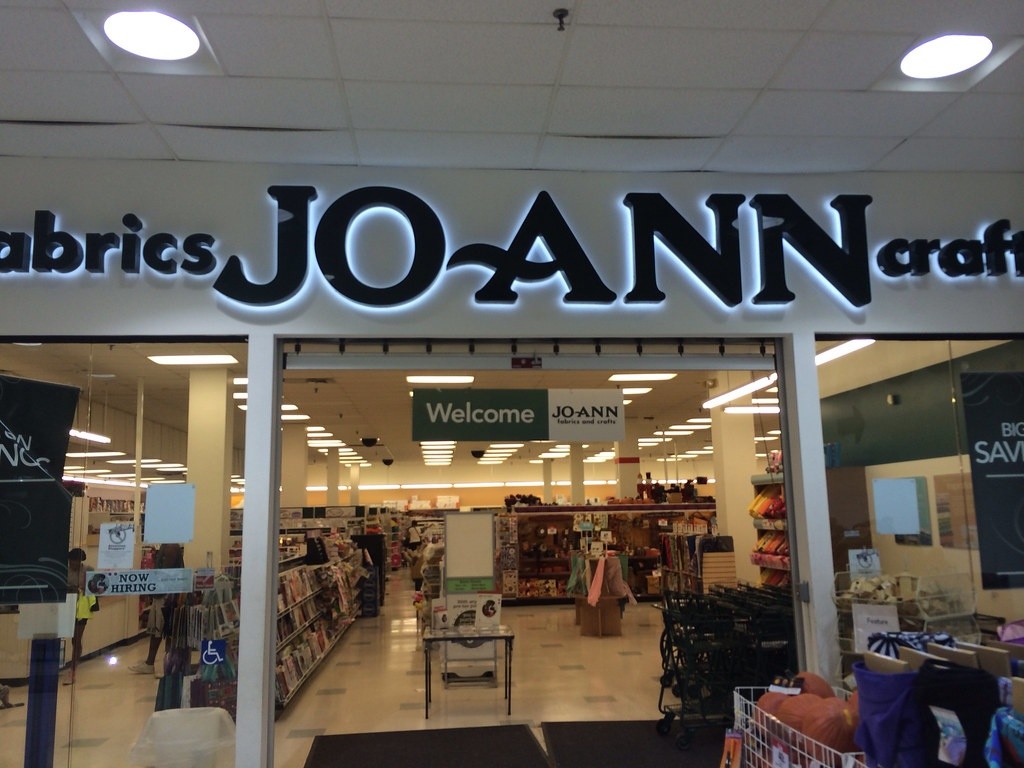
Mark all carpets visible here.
[540,717,742,768]
[303,723,553,768]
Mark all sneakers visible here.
[155,672,165,679]
[128,662,155,674]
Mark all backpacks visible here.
[914,658,1000,768]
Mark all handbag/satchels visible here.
[850,661,933,768]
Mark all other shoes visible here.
[62,670,76,685]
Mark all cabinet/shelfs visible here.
[415,469,791,664]
[167,516,400,712]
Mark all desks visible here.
[422,623,516,720]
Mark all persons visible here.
[408,521,423,551]
[128,542,183,674]
[61,547,96,686]
